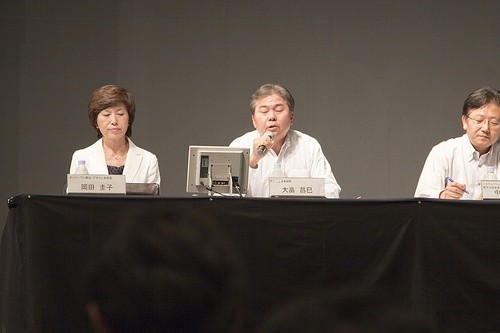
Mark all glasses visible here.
[467,116,499,129]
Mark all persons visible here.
[228,84,341,198]
[66,84,161,195]
[413,85,500,200]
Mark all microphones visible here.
[258,131,274,155]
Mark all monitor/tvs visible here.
[186,145,250,194]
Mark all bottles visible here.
[485,166,497,180]
[75,160,88,174]
[272,164,283,177]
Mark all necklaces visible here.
[103,138,126,160]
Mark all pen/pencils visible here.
[446,176,470,194]
